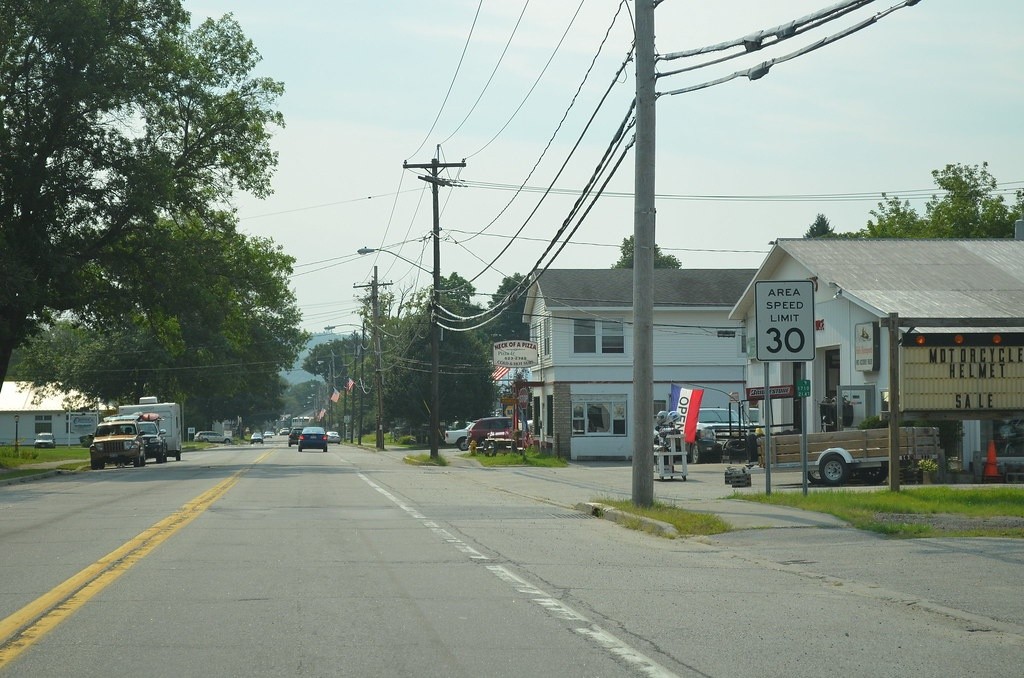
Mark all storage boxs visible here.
[725,471,744,484]
[732,473,751,488]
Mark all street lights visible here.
[14,411,20,458]
[318,359,348,442]
[358,246,441,461]
[324,324,385,450]
[303,368,332,432]
[325,339,355,445]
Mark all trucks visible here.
[118,397,183,462]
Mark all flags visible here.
[316,407,327,422]
[330,388,341,403]
[669,381,705,443]
[345,378,355,393]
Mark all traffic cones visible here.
[983,439,1002,478]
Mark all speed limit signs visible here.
[755,280,815,362]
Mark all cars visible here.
[326,431,341,444]
[33,432,56,449]
[279,427,289,436]
[263,431,272,438]
[271,432,276,436]
[250,432,264,445]
[297,426,328,452]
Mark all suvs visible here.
[465,416,530,454]
[89,420,145,470]
[287,427,304,448]
[137,421,167,463]
[677,407,759,464]
[193,431,234,444]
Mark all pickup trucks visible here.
[444,423,475,451]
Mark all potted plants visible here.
[918,459,938,485]
